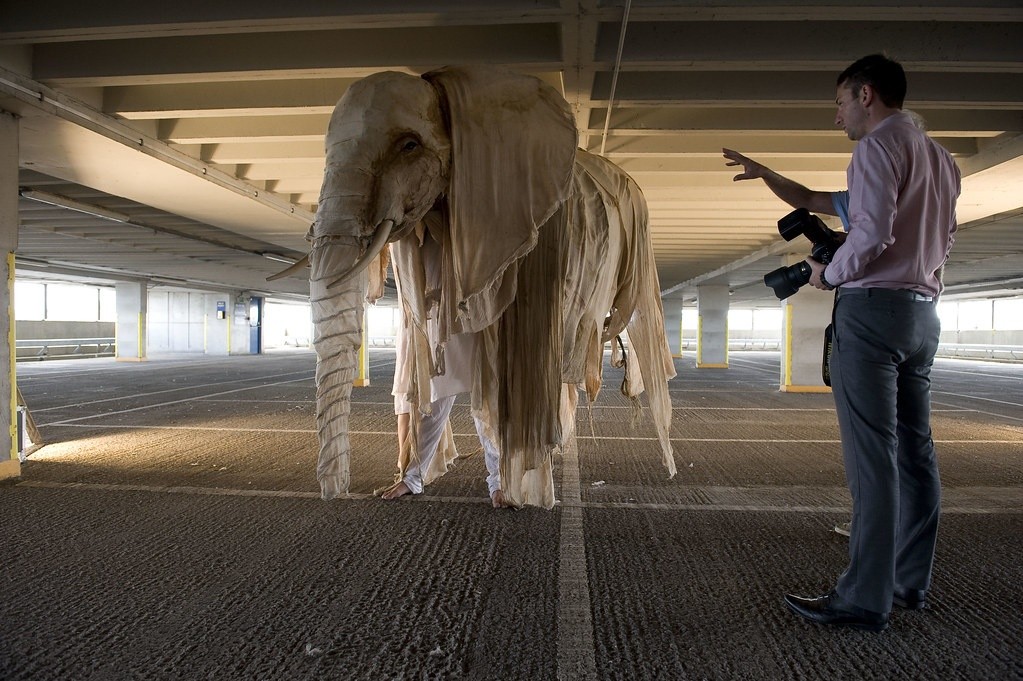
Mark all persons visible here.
[781,52,961,632]
[722,108,927,538]
[381,227,517,509]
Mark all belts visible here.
[838,287,933,302]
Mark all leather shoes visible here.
[784,587,890,629]
[892,582,925,608]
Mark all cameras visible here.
[764,208,848,300]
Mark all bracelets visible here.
[819,267,834,290]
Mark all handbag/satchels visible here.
[821,323,834,387]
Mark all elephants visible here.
[265,64,649,502]
